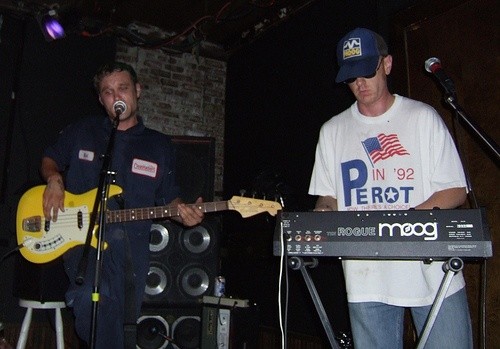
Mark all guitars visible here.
[16,183,282,264]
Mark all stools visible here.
[16,298,66,349]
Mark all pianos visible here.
[272,208,493,349]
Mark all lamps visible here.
[36,2,79,43]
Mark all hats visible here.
[335,27,388,83]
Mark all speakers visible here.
[143,218,222,297]
[165,135,216,217]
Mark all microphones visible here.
[425,58,456,101]
[113,100,127,115]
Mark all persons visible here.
[42,61,202,349]
[308,28,474,349]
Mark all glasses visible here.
[345,56,384,83]
